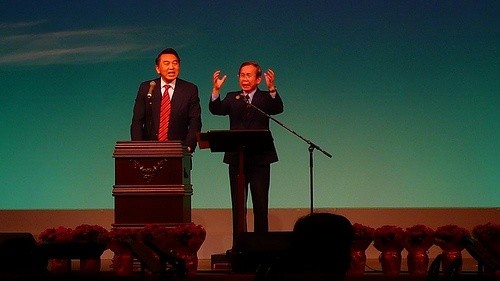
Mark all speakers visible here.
[232,232,292,272]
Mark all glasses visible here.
[237,73,256,78]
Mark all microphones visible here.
[236,93,245,100]
[147,81,156,98]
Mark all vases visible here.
[48,251,463,273]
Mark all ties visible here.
[245,95,250,103]
[158,85,171,142]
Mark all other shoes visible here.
[226,249,240,256]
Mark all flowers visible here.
[35,221,500,253]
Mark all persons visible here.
[209,62,284,250]
[130,48,203,153]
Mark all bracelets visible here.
[269,88,277,93]
